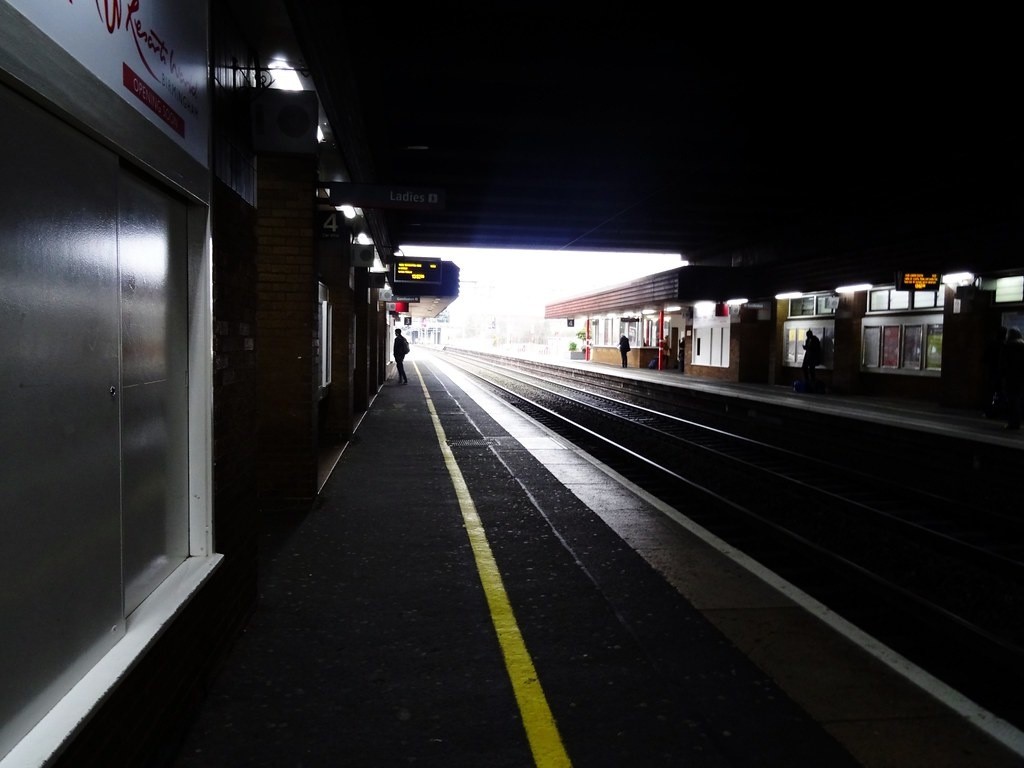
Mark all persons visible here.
[663,335,671,369]
[1003,328,1024,429]
[979,326,1007,418]
[679,337,685,372]
[394,329,407,384]
[802,330,820,393]
[619,334,629,368]
